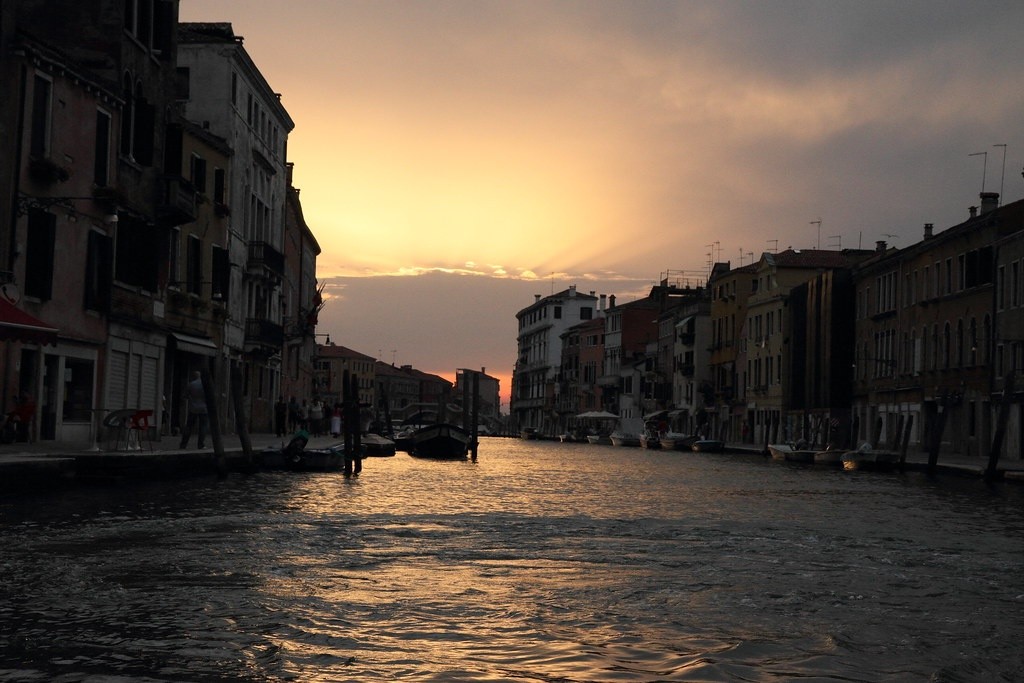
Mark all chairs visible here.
[99,409,143,453]
[125,409,153,453]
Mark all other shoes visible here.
[199,447,206,450]
[179,446,186,450]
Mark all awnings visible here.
[667,409,688,418]
[0,296,60,348]
[643,411,665,420]
[171,332,218,358]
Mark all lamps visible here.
[281,333,331,345]
[14,196,118,225]
[160,279,222,302]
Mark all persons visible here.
[162,395,166,436]
[299,398,310,432]
[179,370,209,450]
[288,396,298,434]
[274,396,287,437]
[310,399,376,438]
[5,395,32,445]
[700,421,712,440]
[741,421,748,444]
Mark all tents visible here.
[574,410,621,428]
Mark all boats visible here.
[767,443,842,464]
[587,436,610,445]
[660,435,700,451]
[918,461,986,489]
[692,440,721,452]
[359,432,396,457]
[638,435,660,449]
[392,420,471,458]
[840,449,901,471]
[609,436,640,447]
[521,428,541,441]
